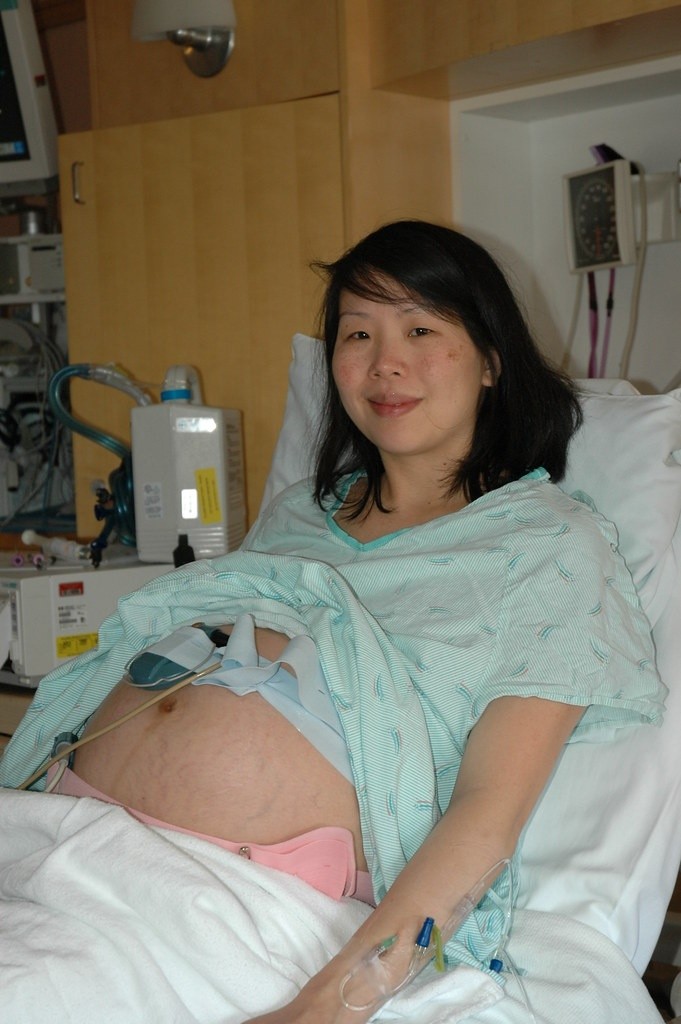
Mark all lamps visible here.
[130,0,237,77]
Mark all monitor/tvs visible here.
[0,0,61,197]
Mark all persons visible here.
[0,221,671,1024]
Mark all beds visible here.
[0,376,681,1024]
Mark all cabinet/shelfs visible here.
[57,0,451,541]
[365,0,681,102]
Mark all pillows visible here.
[258,332,681,631]
